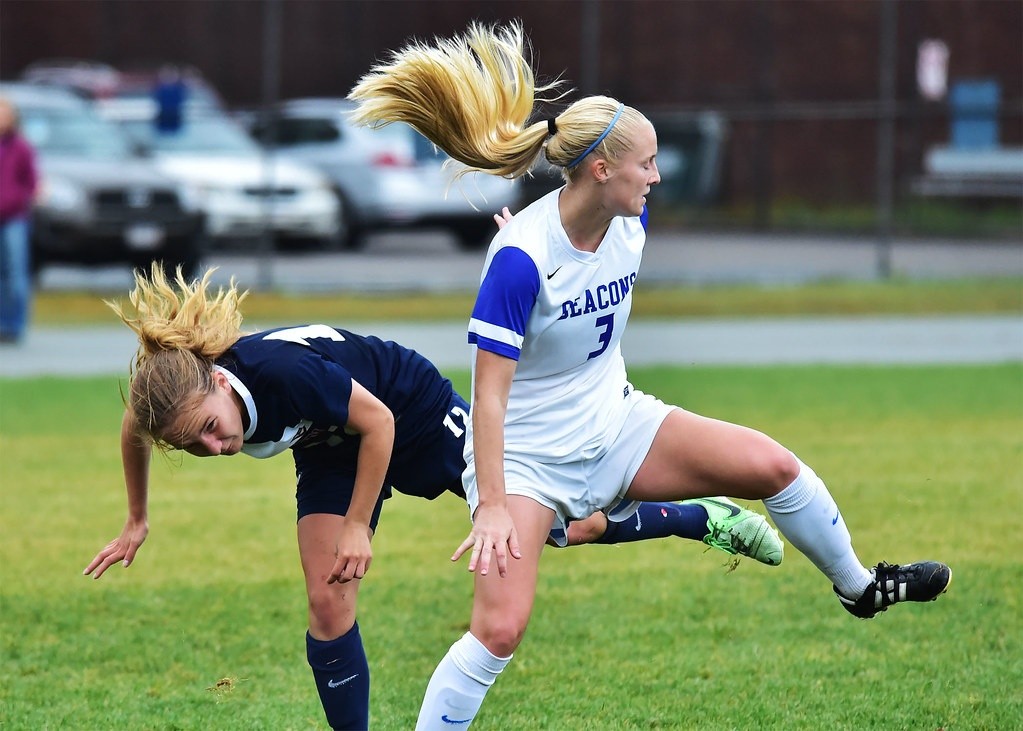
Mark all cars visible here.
[80,80,346,255]
[0,82,211,286]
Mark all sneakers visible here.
[833,560,952,619]
[679,496,784,567]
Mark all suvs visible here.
[240,93,527,254]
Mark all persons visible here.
[336,17,952,731]
[0,97,40,345]
[79,262,784,731]
